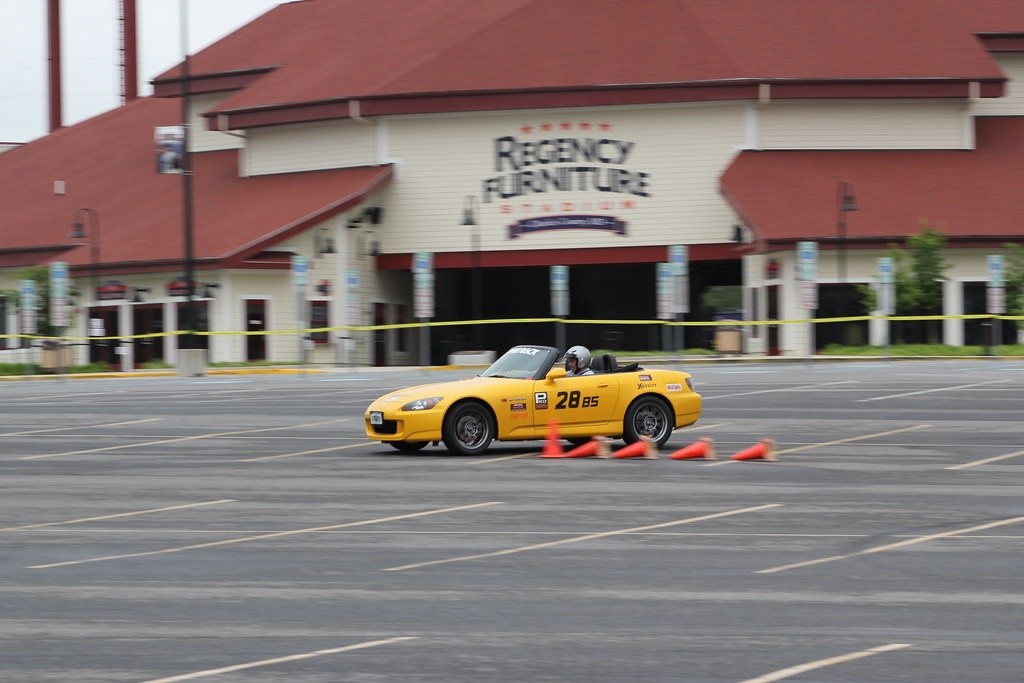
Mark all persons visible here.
[563,346,595,377]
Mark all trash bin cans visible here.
[40,339,67,367]
[714,320,744,358]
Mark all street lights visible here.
[835,179,865,347]
[66,207,100,363]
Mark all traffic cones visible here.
[563,434,613,458]
[609,435,658,458]
[538,417,565,459]
[666,438,717,460]
[728,438,779,462]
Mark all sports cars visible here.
[363,344,703,455]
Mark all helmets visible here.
[563,346,591,369]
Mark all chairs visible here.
[565,351,617,374]
